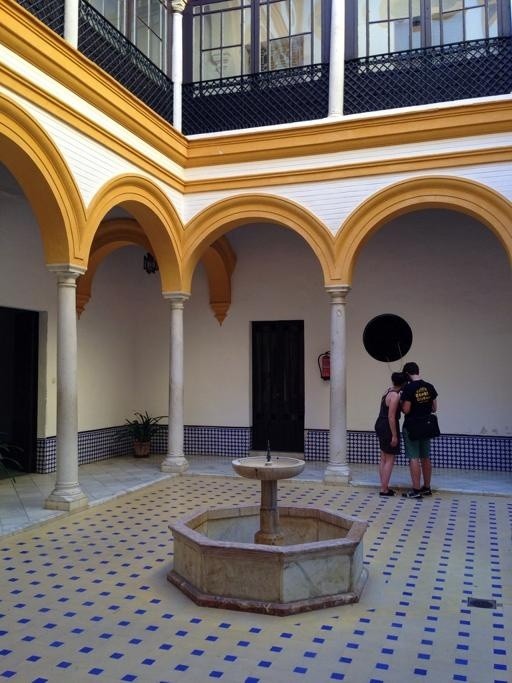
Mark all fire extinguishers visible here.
[317,351,330,379]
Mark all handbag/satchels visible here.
[406,414,440,441]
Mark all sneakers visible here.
[402,491,422,498]
[420,486,432,495]
[378,489,396,497]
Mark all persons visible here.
[401,362,438,497]
[374,372,408,496]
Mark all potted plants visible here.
[111,408,169,457]
